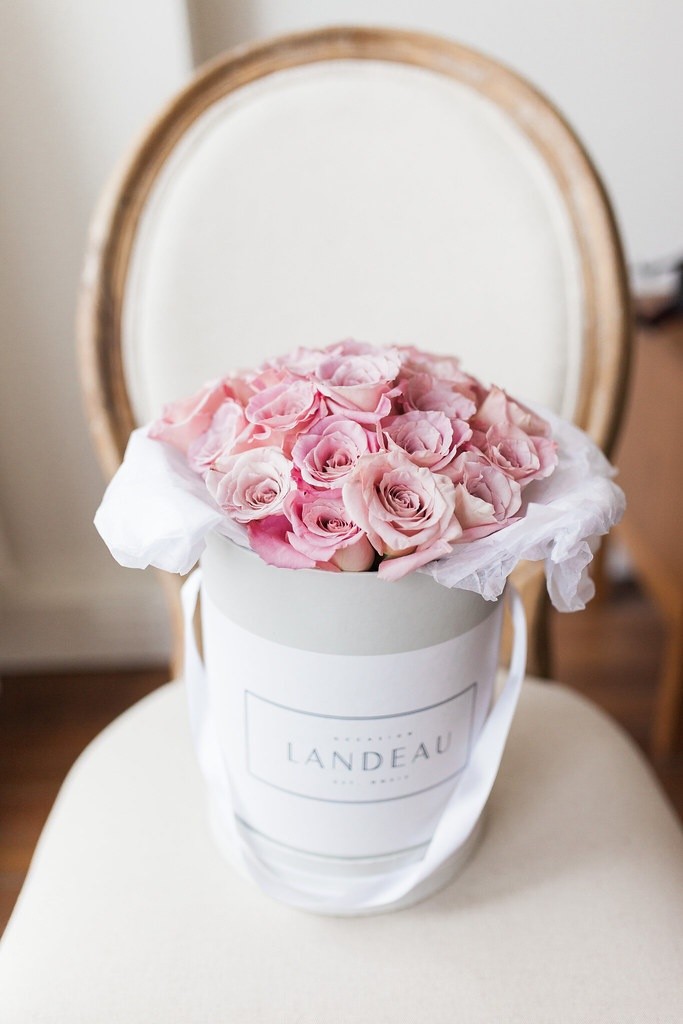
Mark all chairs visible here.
[0,27,683,1024]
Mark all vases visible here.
[195,532,515,911]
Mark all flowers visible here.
[150,336,558,585]
